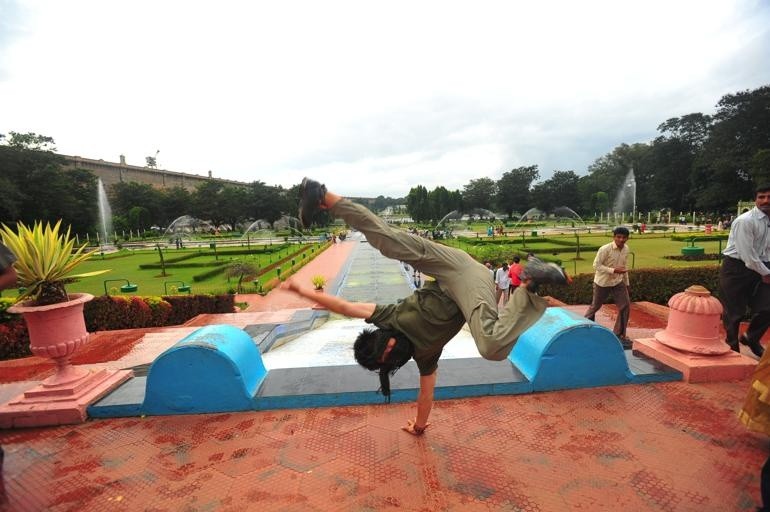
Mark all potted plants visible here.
[0,213,113,388]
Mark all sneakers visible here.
[518,255,568,289]
[617,335,634,349]
[298,176,327,228]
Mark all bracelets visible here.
[413,422,425,433]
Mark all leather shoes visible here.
[739,335,765,357]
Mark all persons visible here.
[487,223,504,236]
[414,277,421,289]
[179,238,182,248]
[274,177,572,435]
[720,182,770,357]
[0,241,19,290]
[406,224,452,277]
[584,228,634,347]
[655,213,735,234]
[641,222,646,233]
[485,253,534,319]
[176,238,180,249]
[332,232,336,244]
